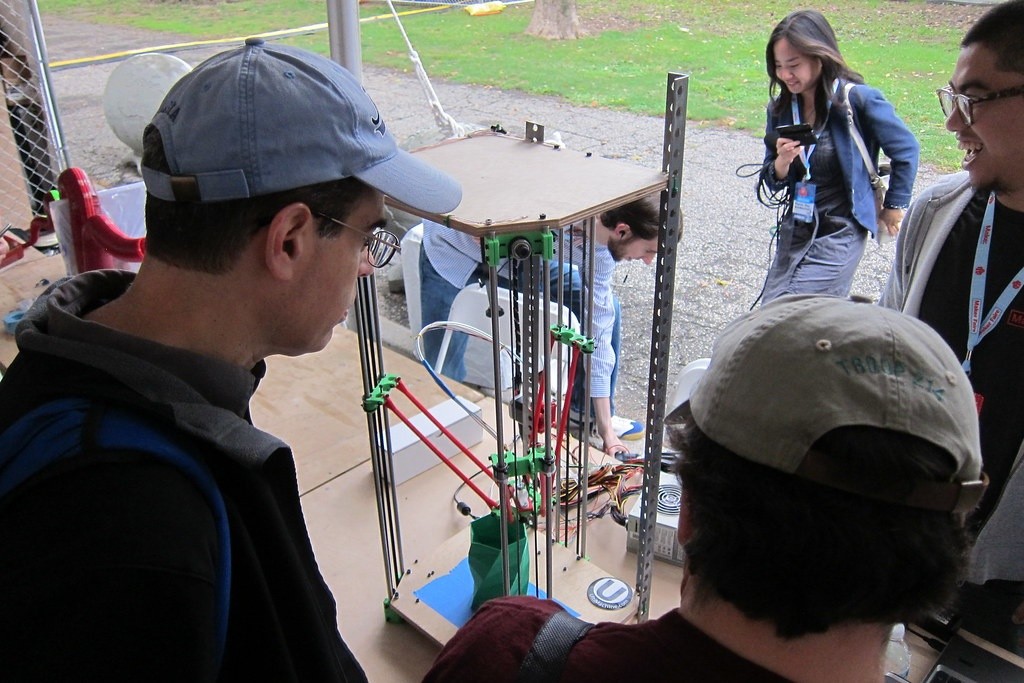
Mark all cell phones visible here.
[777,124,818,147]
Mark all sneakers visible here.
[565,409,644,440]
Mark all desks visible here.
[356,74,689,656]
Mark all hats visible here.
[143,38,462,214]
[663,293,989,512]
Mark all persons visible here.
[754,9,920,304]
[878,0,1024,658]
[0,36,464,683]
[418,290,990,683]
[420,189,684,463]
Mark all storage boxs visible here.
[373,392,485,486]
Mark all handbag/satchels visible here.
[845,84,902,246]
[466,510,530,610]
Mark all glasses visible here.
[937,86,1024,125]
[309,210,402,268]
